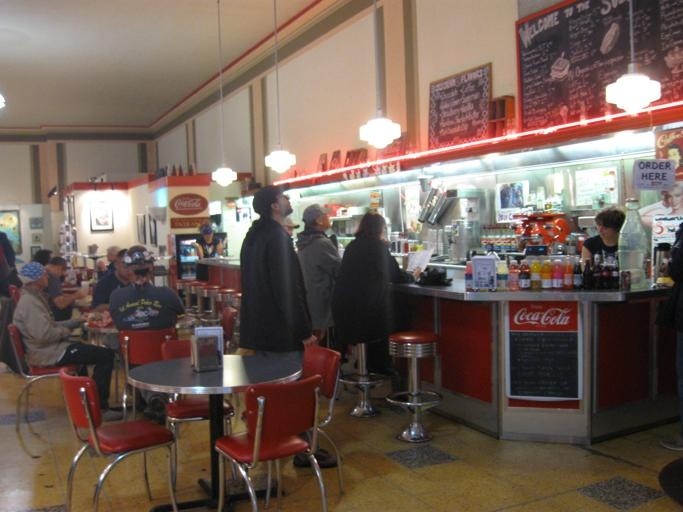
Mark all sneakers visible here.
[660,437,683,452]
[293,448,344,467]
[101,409,121,421]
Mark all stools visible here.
[388,330,442,442]
[338,326,391,418]
[175,278,242,326]
[317,325,344,378]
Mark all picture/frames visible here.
[0,209,24,256]
[61,194,75,226]
[90,207,114,231]
[28,217,44,258]
[135,212,157,247]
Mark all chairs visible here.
[7,284,20,302]
[116,326,179,420]
[265,344,348,491]
[9,324,75,429]
[219,306,238,355]
[53,367,179,511]
[215,374,330,511]
[158,332,235,488]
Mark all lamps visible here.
[357,2,402,148]
[603,0,661,116]
[47,185,59,197]
[261,1,299,175]
[211,0,238,189]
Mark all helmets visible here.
[123,246,154,265]
[200,225,212,235]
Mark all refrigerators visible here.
[165,232,199,296]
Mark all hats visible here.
[302,204,331,224]
[18,261,47,284]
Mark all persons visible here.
[330,211,423,381]
[108,245,186,424]
[579,207,625,265]
[92,247,134,351]
[238,184,344,470]
[281,215,301,236]
[191,227,226,308]
[657,213,682,453]
[637,183,683,225]
[1,234,19,296]
[12,263,114,411]
[295,203,343,347]
[33,250,89,323]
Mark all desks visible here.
[83,307,217,409]
[126,352,304,511]
[58,248,174,312]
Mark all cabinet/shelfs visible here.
[329,213,417,255]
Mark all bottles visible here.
[614,197,647,285]
[463,259,620,292]
